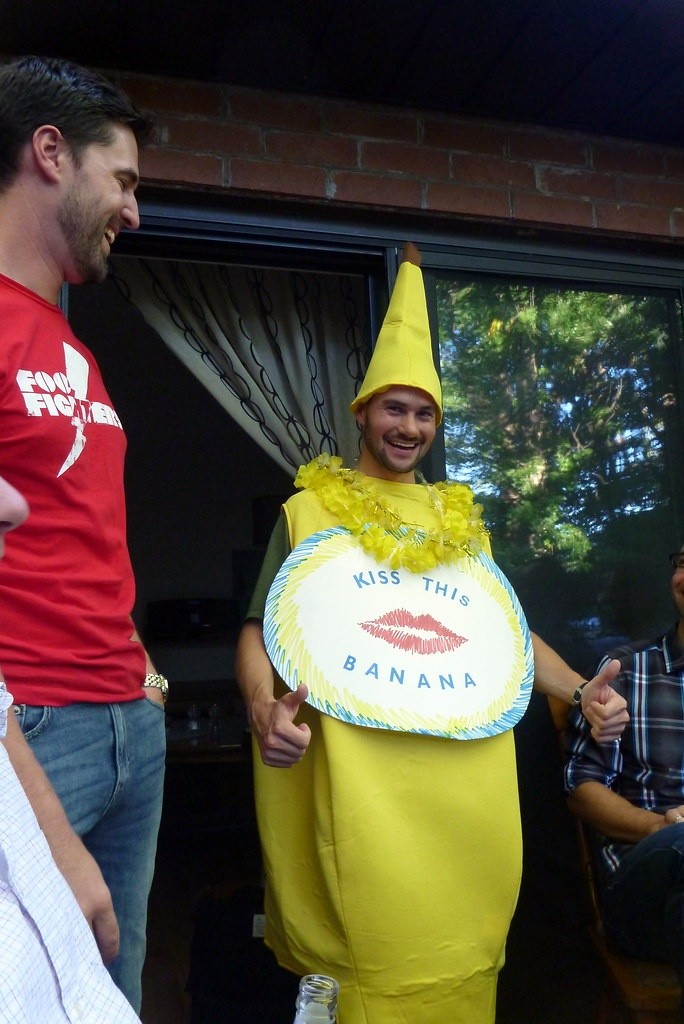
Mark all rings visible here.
[672,815,683,824]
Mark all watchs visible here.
[141,673,170,704]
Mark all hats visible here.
[352,242,443,425]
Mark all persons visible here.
[0,476,143,1024]
[556,541,684,989]
[231,241,632,1023]
[0,55,169,1024]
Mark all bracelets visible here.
[572,680,592,714]
